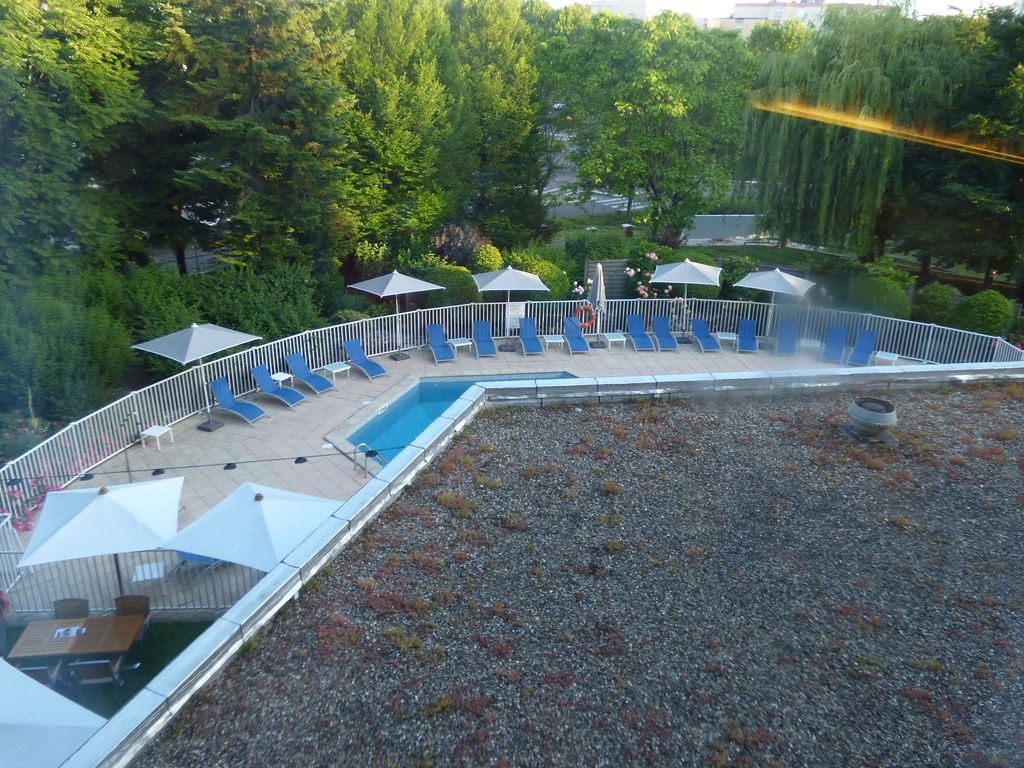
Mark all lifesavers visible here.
[574,305,595,327]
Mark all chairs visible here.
[691,319,723,355]
[210,378,271,428]
[251,365,312,412]
[471,321,498,359]
[517,317,545,357]
[820,327,877,367]
[735,319,759,355]
[651,316,680,354]
[342,339,391,383]
[625,315,656,353]
[426,324,458,366]
[774,320,798,355]
[285,354,339,397]
[14,595,153,699]
[563,317,591,356]
[161,548,228,596]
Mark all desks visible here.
[875,351,899,367]
[543,335,564,351]
[7,615,143,695]
[799,339,820,356]
[603,333,626,349]
[141,425,174,451]
[446,337,472,354]
[322,362,351,381]
[271,372,294,389]
[716,332,736,349]
[131,562,165,597]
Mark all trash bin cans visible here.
[622,224,635,237]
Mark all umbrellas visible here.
[649,258,722,344]
[470,265,549,352]
[158,481,345,574]
[132,323,263,432]
[17,477,185,568]
[587,262,607,349]
[733,268,816,350]
[0,657,108,768]
[347,270,446,361]
